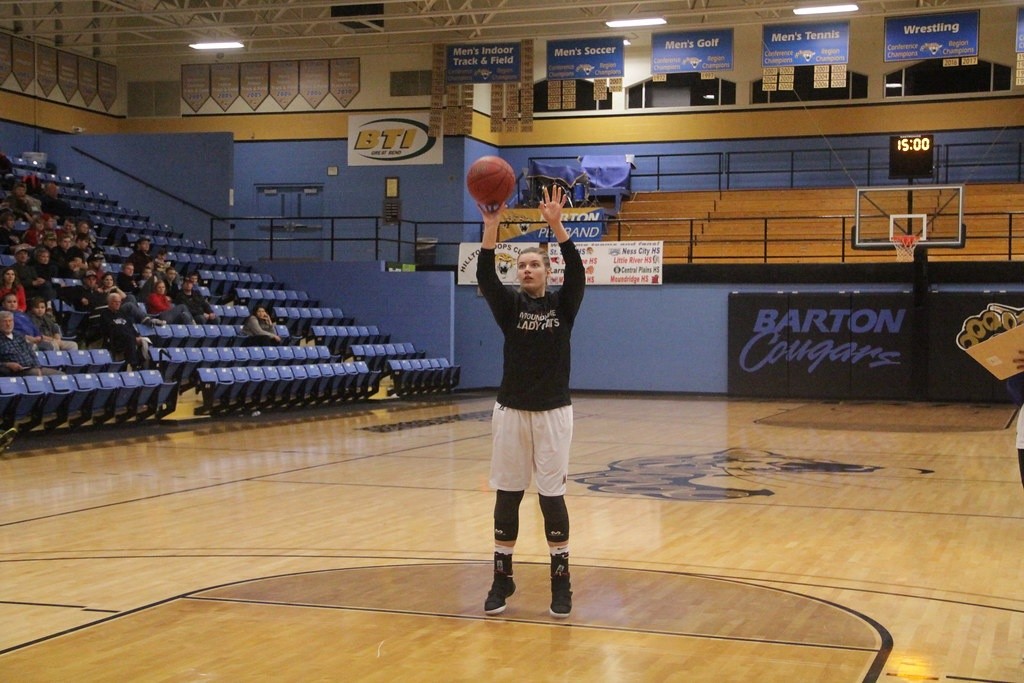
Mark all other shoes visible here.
[143,317,154,328]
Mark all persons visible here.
[0,153,221,376]
[473,184,587,618]
[245,305,282,346]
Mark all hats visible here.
[136,237,152,245]
[44,231,57,241]
[84,269,97,279]
[15,246,27,256]
[90,254,105,260]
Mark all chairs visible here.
[0,157,462,434]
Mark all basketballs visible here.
[466,154,516,206]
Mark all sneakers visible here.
[550,573,573,618]
[484,572,516,615]
